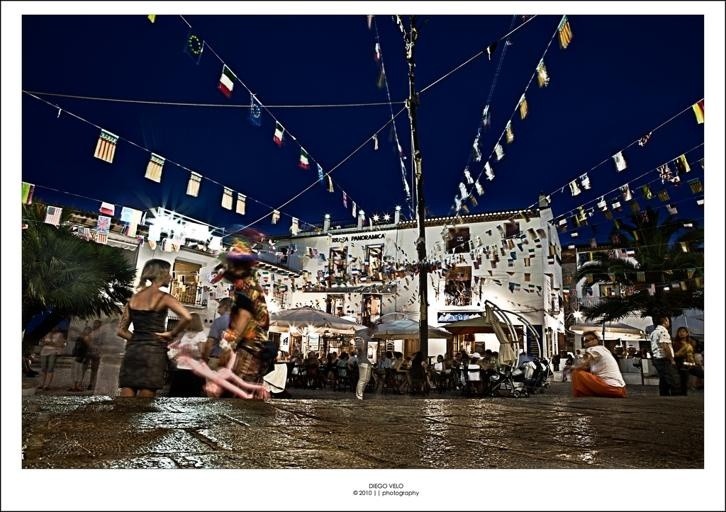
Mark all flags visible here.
[23,14,705,311]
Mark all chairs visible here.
[289,360,450,396]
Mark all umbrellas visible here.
[272,305,358,349]
[362,316,449,351]
[443,315,521,333]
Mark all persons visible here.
[565,331,631,397]
[650,314,680,396]
[353,321,375,400]
[575,350,583,358]
[36,241,271,401]
[290,350,547,399]
[673,326,697,395]
